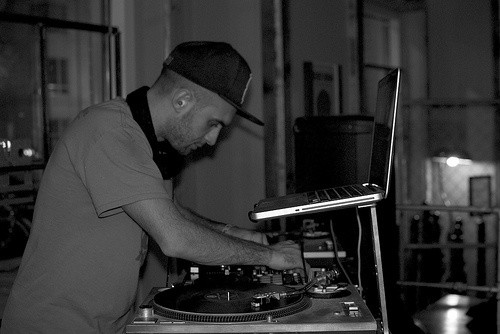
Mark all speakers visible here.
[293,114,375,239]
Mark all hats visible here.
[163,41,265,127]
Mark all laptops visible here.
[248,68,400,221]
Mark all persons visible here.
[1,40,311,334]
